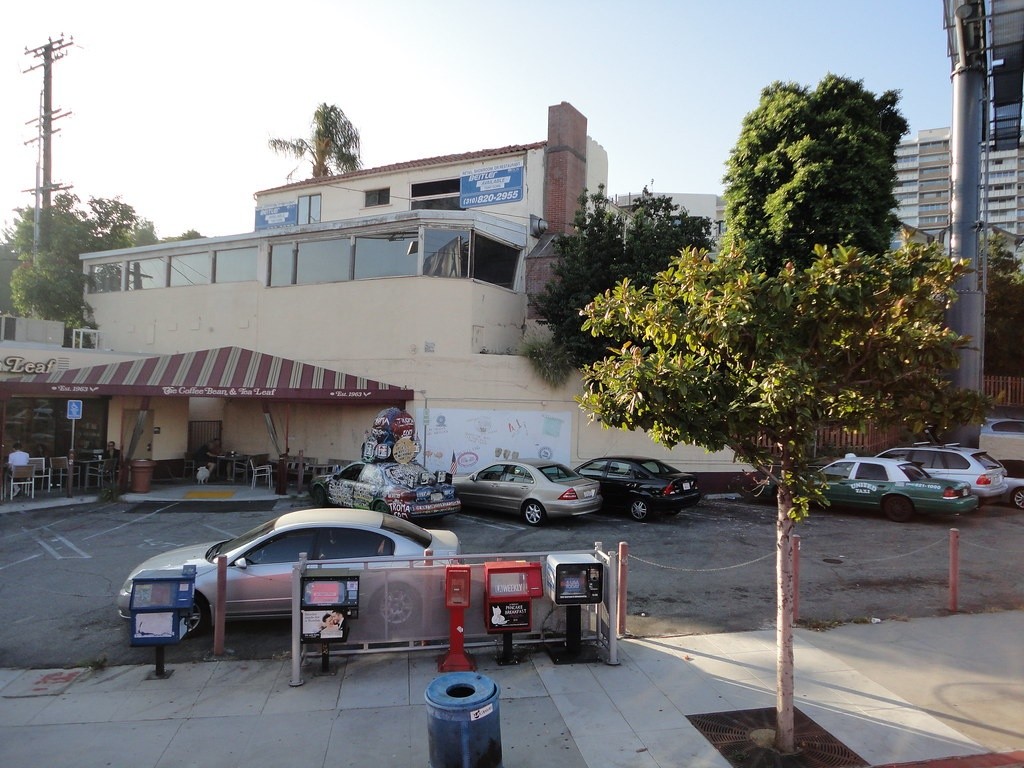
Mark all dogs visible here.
[196,463,215,484]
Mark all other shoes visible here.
[13,486,20,496]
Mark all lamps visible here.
[407,240,459,256]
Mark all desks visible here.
[304,464,340,475]
[76,457,115,493]
[216,455,248,481]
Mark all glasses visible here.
[108,445,113,447]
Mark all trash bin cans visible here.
[130,458,157,494]
[425,671,503,768]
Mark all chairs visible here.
[184,450,351,490]
[610,463,620,473]
[624,469,636,477]
[0,445,124,503]
[499,465,515,482]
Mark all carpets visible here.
[182,490,235,499]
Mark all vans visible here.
[979,416,1024,512]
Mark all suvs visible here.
[845,442,1007,508]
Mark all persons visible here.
[319,610,349,638]
[196,438,223,481]
[7,443,51,497]
[105,441,122,484]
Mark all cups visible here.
[231,451,234,454]
[98,455,102,460]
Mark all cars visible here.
[118,505,462,641]
[572,455,703,522]
[308,457,462,524]
[450,458,605,525]
[800,452,981,523]
